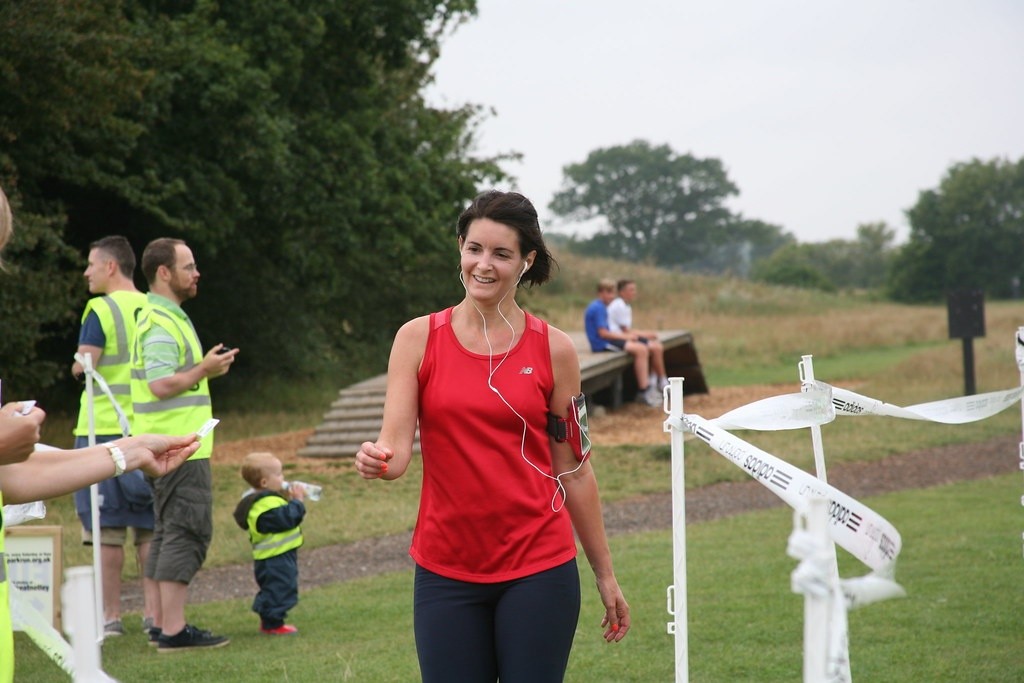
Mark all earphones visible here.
[519,261,528,276]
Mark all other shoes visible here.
[142,617,155,632]
[258,622,298,637]
[644,374,670,408]
[104,620,124,635]
[148,627,231,654]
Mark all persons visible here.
[127,237,240,653]
[584,276,664,409]
[233,451,307,636]
[71,236,159,640]
[354,187,632,683]
[605,277,671,394]
[0,187,202,682]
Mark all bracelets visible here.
[100,440,127,478]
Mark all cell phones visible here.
[577,401,591,454]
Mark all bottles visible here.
[281,481,322,501]
